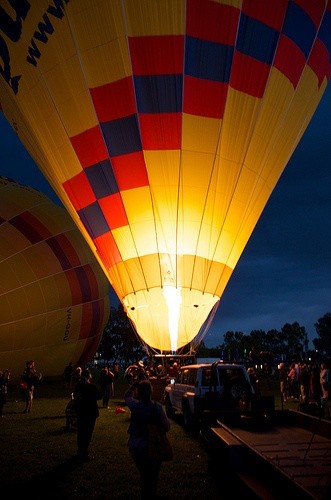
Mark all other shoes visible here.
[24,409,28,414]
[78,455,89,462]
[28,409,31,413]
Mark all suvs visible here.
[162,360,256,428]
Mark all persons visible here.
[125,380,170,500]
[1,358,330,462]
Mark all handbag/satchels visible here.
[152,401,173,461]
[20,382,28,390]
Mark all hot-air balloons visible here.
[0,175,111,387]
[1,0,331,404]
